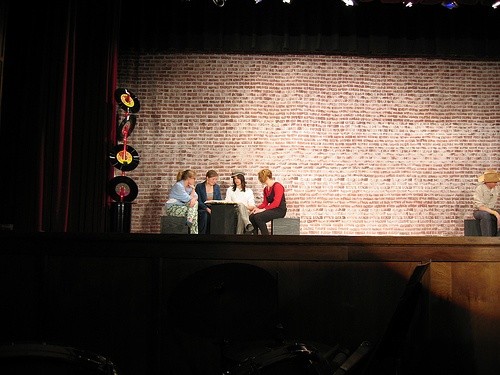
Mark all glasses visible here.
[190,177,195,180]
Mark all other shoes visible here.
[185,221,192,227]
[246,224,254,231]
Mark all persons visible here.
[195,170,222,234]
[249,169,287,235]
[473,170,500,236]
[165,169,199,234]
[224,171,257,235]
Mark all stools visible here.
[464,216,482,236]
[271,218,300,236]
[160,216,191,233]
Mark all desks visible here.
[204,200,239,233]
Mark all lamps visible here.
[488,0,500,9]
[343,0,355,6]
[214,0,227,7]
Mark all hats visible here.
[478,172,500,184]
[231,172,245,178]
